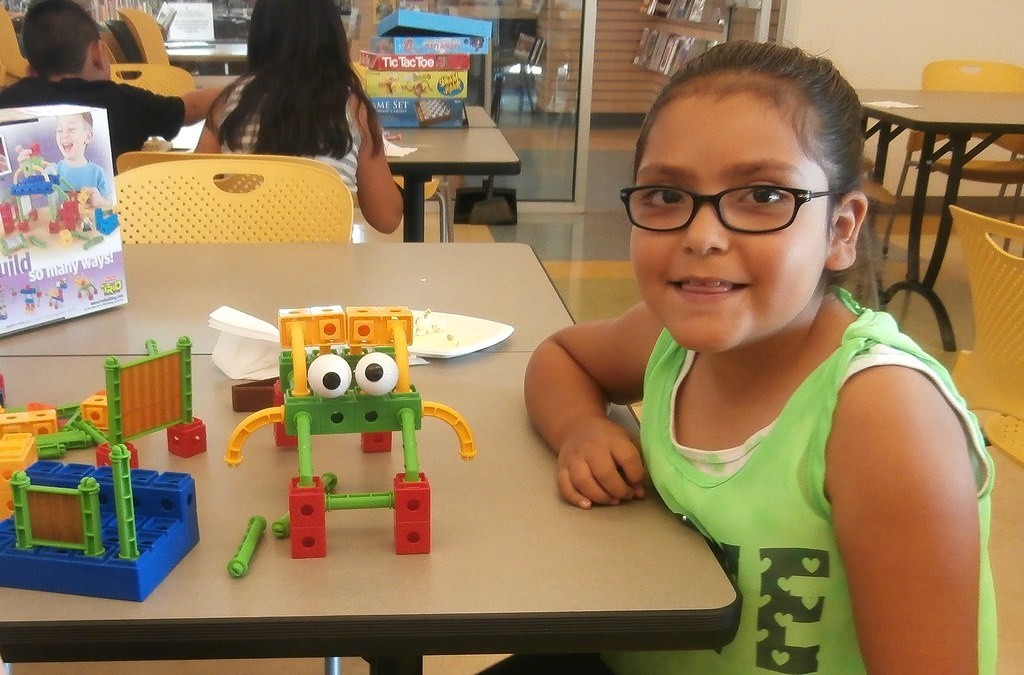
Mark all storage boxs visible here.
[352,61,467,98]
[371,9,493,56]
[368,98,463,128]
[359,49,470,71]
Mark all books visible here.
[632,0,705,77]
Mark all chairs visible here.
[99,32,126,64]
[108,63,195,98]
[106,20,142,64]
[117,7,171,67]
[883,60,1024,253]
[949,204,1024,470]
[115,159,353,244]
[0,4,30,80]
[115,151,342,183]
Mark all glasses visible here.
[620,185,843,233]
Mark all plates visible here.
[407,310,514,360]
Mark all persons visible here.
[193,0,403,243]
[474,40,999,675]
[43,112,112,209]
[1,0,226,156]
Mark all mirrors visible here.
[375,0,598,212]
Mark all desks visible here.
[0,241,743,662]
[463,105,497,127]
[853,88,1024,351]
[142,127,522,243]
